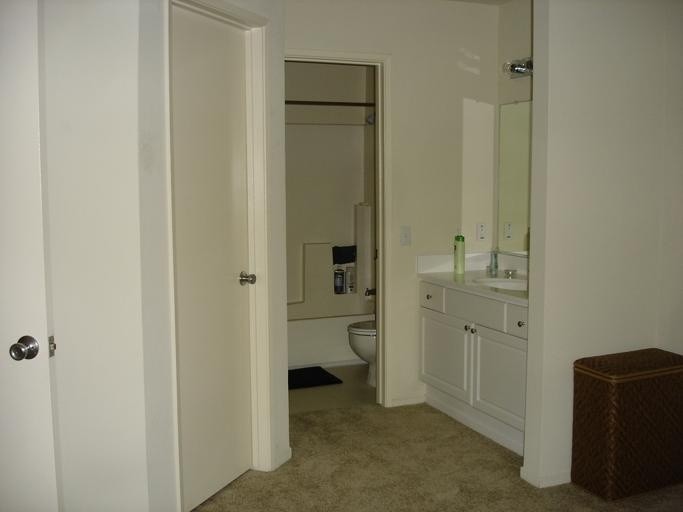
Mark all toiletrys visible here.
[485,251,498,278]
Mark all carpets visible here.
[288,366,343,390]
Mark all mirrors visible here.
[497,100,532,258]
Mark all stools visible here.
[571,348,683,501]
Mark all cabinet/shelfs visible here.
[419,281,528,458]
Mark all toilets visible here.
[347,320,377,388]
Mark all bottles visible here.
[334,266,344,294]
[345,267,355,293]
[453,235,466,275]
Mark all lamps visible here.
[503,57,533,79]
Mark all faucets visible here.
[365,288,376,296]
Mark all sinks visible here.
[476,278,528,292]
